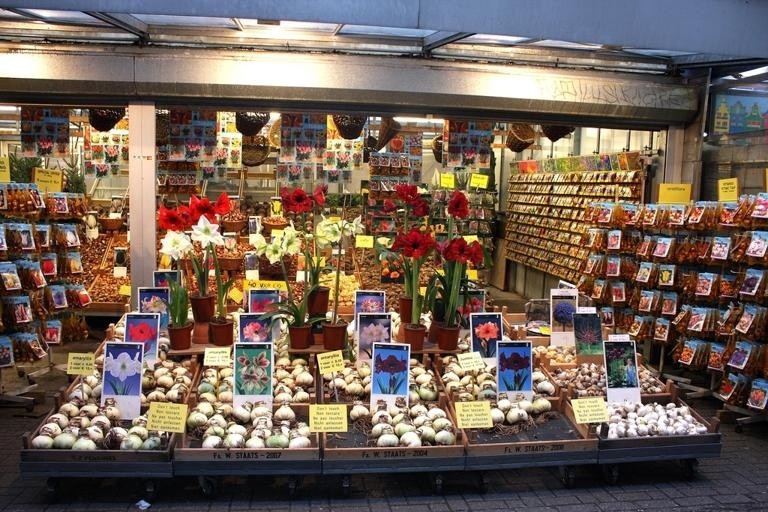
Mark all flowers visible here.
[272,138,363,181]
[23,110,129,175]
[156,109,241,179]
